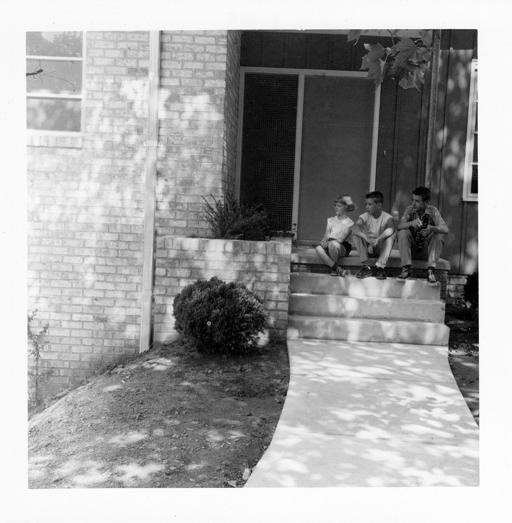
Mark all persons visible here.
[350,190,397,279]
[396,185,449,283]
[314,193,357,276]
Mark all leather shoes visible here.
[336,265,346,276]
[330,269,338,275]
[374,265,386,279]
[357,265,374,278]
[427,269,437,283]
[396,269,411,282]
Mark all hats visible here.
[336,194,355,211]
